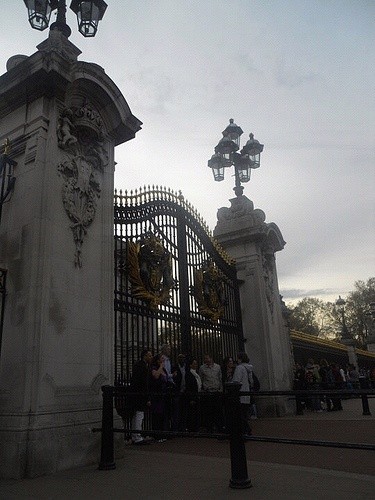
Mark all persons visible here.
[199,352,225,431]
[232,352,255,437]
[128,347,152,445]
[248,371,259,420]
[294,357,375,413]
[161,343,176,385]
[173,353,189,396]
[221,357,236,388]
[188,358,202,405]
[151,355,175,442]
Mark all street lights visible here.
[336,295,353,339]
[207,119,264,198]
[23,0,109,40]
[369,301,375,318]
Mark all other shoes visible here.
[249,415,257,420]
[131,433,253,444]
[298,401,343,417]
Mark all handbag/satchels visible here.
[332,367,344,386]
[306,371,318,384]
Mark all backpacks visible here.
[249,372,260,390]
